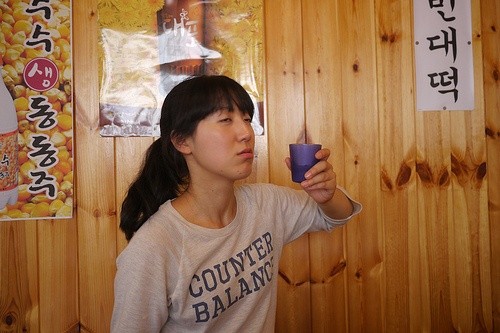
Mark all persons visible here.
[111,74,364,333]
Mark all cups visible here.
[288,143,323,183]
